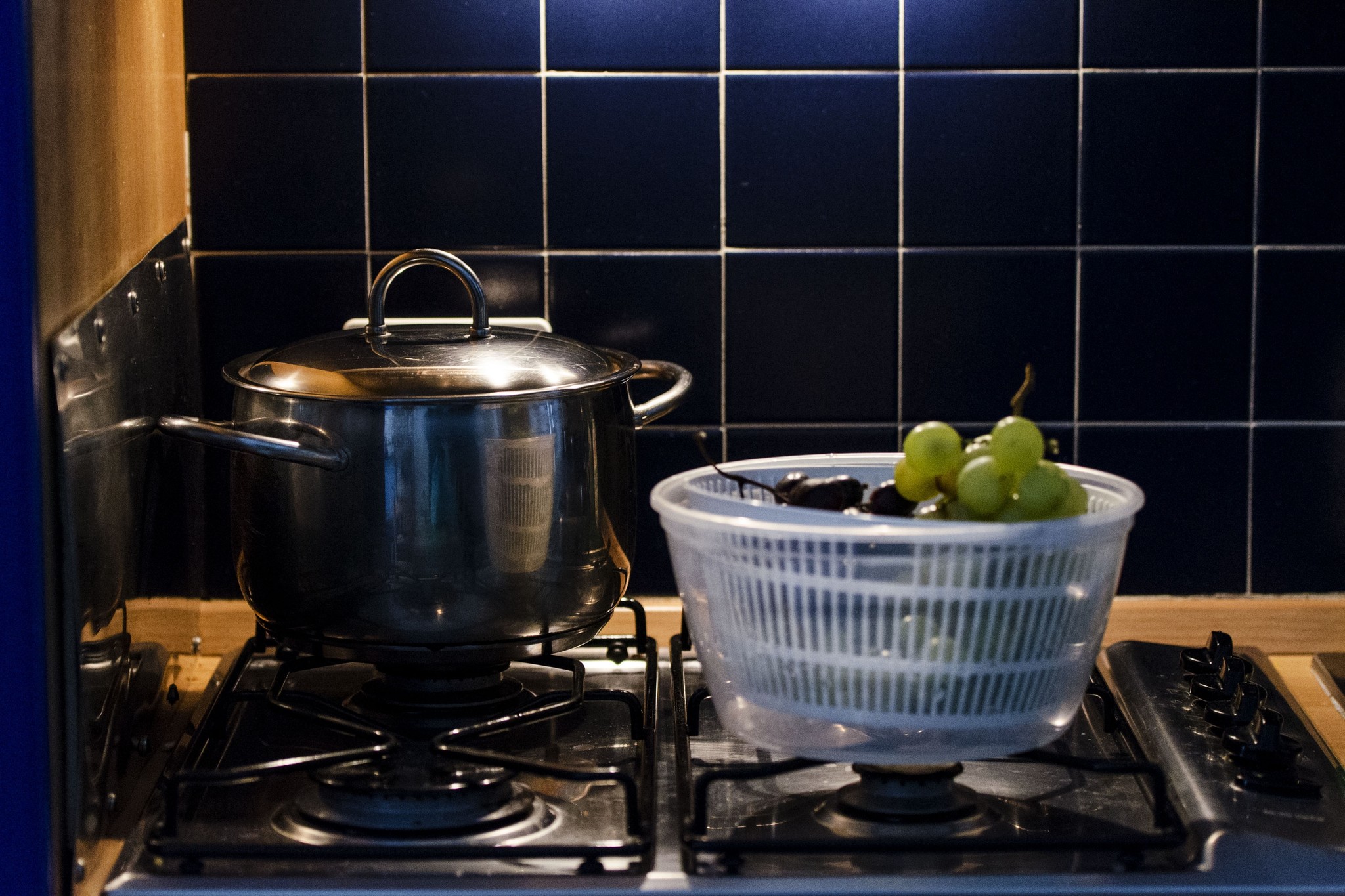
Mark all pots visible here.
[157,243,692,659]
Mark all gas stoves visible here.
[102,628,1344,894]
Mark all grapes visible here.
[763,415,1094,689]
